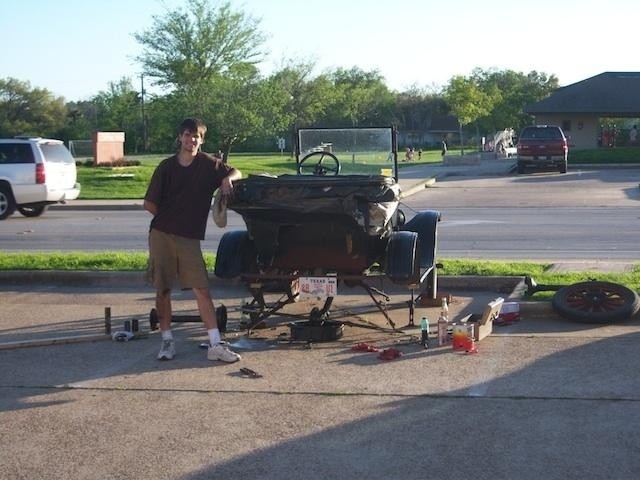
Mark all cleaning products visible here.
[438,312,448,346]
[440,297,447,316]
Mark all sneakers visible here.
[157,337,177,361]
[206,340,241,363]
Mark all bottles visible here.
[419,317,431,346]
[437,312,449,348]
[439,297,449,321]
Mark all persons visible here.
[440,141,448,157]
[386,152,394,161]
[630,125,638,140]
[403,148,423,162]
[142,116,242,365]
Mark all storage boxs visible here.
[460,297,504,341]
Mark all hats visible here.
[213,189,227,228]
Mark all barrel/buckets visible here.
[452,323,475,354]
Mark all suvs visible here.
[515,124,571,175]
[0,136,82,222]
